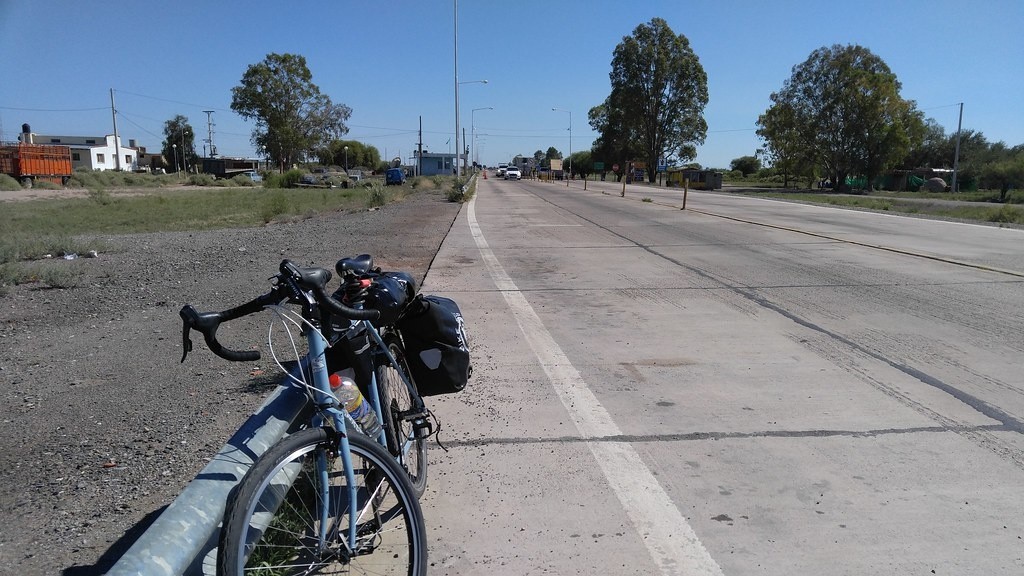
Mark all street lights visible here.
[552,108,572,178]
[172,144,178,173]
[182,131,189,179]
[262,145,266,158]
[472,107,494,176]
[455,79,489,185]
[344,146,349,175]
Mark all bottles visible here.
[330,374,383,440]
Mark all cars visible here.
[386,169,406,186]
[504,166,522,181]
[244,172,262,183]
[496,164,509,178]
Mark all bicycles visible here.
[178,254,439,576]
